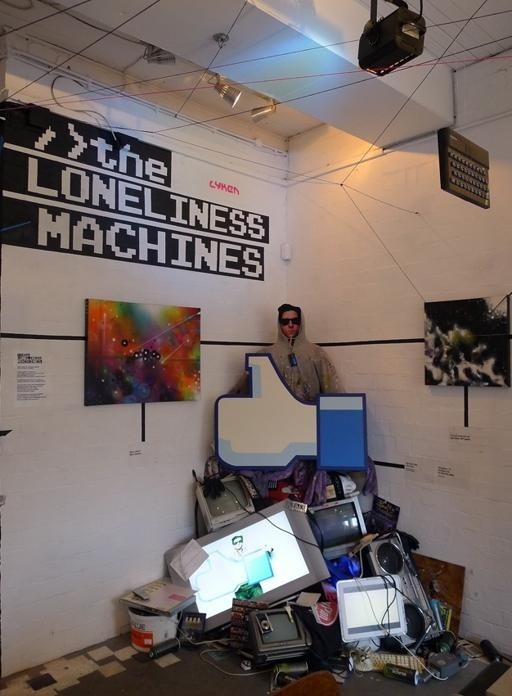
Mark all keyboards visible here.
[353,649,427,675]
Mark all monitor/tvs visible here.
[195,474,256,528]
[306,497,368,562]
[164,498,331,632]
[248,609,310,663]
[335,574,408,650]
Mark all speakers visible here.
[365,538,440,648]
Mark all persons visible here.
[227,304,345,403]
[231,536,263,601]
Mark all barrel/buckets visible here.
[128,607,178,653]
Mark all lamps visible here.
[143,43,278,124]
[356,0,428,80]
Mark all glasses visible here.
[279,318,301,325]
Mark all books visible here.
[121,581,198,616]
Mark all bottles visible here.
[438,631,456,654]
